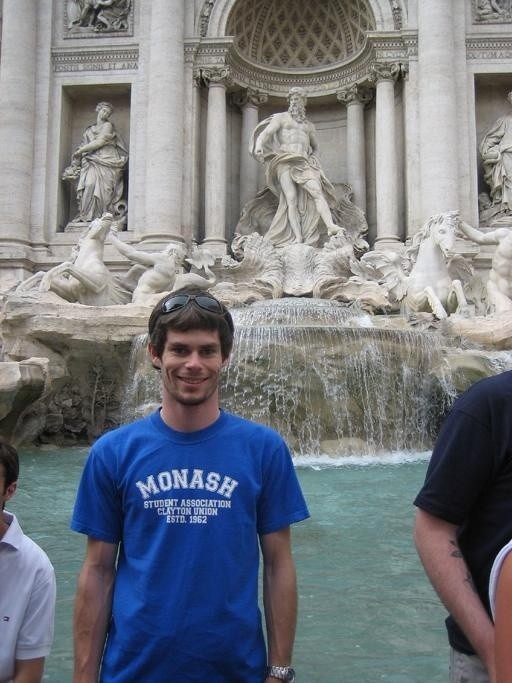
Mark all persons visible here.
[494,543,512,682]
[247,87,347,247]
[107,227,215,310]
[473,0,512,20]
[73,1,133,32]
[478,92,512,223]
[62,103,128,222]
[71,283,308,683]
[412,372,512,682]
[0,443,56,683]
[457,217,512,307]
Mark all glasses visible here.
[162,294,224,314]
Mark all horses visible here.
[16,211,131,304]
[404,208,469,320]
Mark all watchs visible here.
[268,663,293,681]
